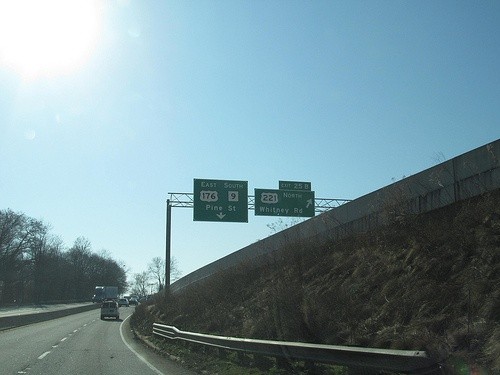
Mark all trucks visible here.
[92,286,119,303]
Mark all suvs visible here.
[129,298,138,305]
[118,298,129,307]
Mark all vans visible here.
[100,301,120,321]
[124,296,132,305]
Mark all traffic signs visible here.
[254,180,316,217]
[193,178,248,223]
